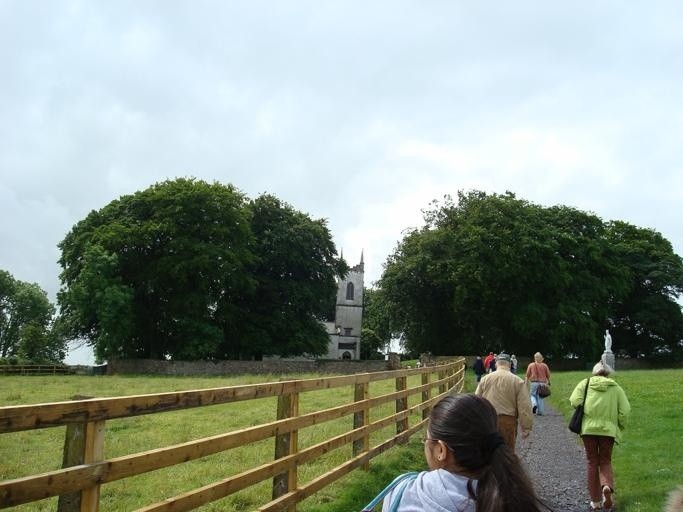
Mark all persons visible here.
[472,352,518,381]
[525,351,551,415]
[568,361,630,510]
[474,353,533,456]
[603,329,612,351]
[381,392,553,511]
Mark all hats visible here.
[495,354,511,362]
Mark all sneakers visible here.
[589,500,601,510]
[602,486,613,512]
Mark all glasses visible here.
[419,436,455,452]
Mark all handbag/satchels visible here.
[537,384,551,398]
[568,404,584,436]
